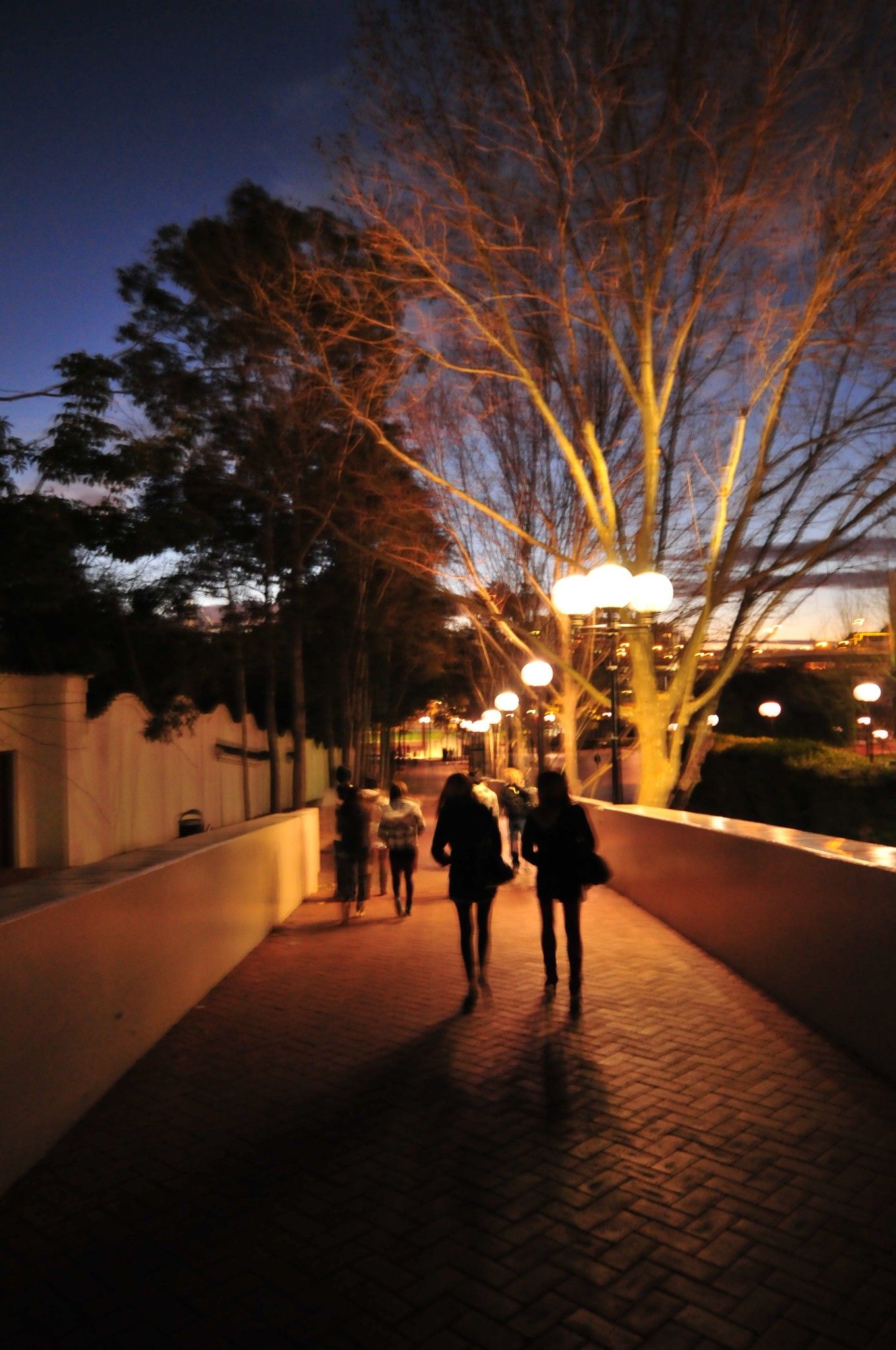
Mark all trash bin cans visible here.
[178,809,204,837]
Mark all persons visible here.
[429,774,504,1009]
[520,773,595,1017]
[319,765,535,926]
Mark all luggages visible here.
[478,975,488,984]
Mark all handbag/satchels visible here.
[485,857,515,884]
[581,853,608,884]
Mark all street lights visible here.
[853,683,881,763]
[520,659,553,776]
[549,563,674,807]
[494,692,520,770]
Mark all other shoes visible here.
[469,987,478,998]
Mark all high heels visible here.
[569,977,581,991]
[545,976,559,988]
